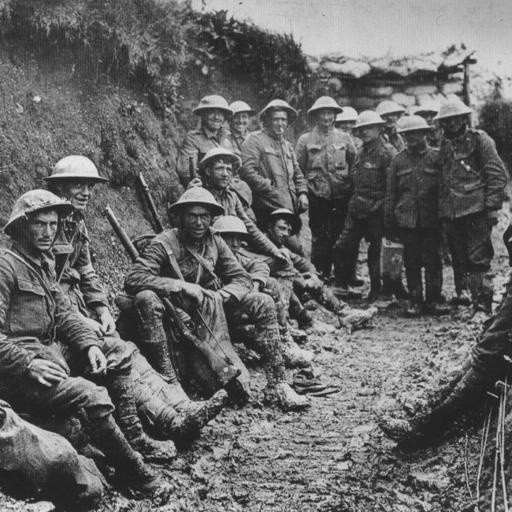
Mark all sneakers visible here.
[116,461,162,489]
[265,306,377,408]
[130,435,178,462]
[323,269,396,307]
[155,408,210,451]
[378,416,414,442]
[404,396,430,418]
[406,282,493,325]
[182,388,230,425]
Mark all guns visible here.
[104,206,240,386]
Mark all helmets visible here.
[199,148,243,176]
[168,187,226,218]
[44,154,111,184]
[271,209,303,237]
[211,216,249,236]
[193,94,473,134]
[3,189,73,237]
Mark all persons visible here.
[37,154,228,448]
[0,399,103,512]
[125,92,376,409]
[332,94,509,312]
[0,189,178,508]
[376,225,512,447]
